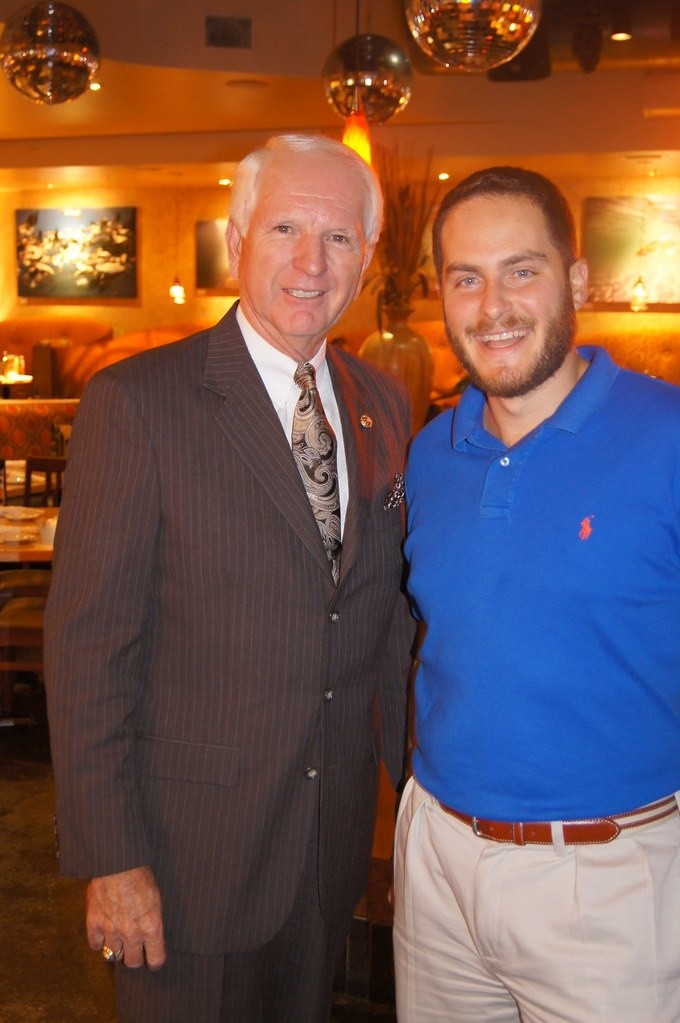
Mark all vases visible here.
[357,308,437,446]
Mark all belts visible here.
[439,795,679,845]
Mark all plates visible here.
[3,506,44,519]
[0,525,39,544]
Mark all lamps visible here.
[325,0,546,127]
[610,13,632,41]
[0,0,100,105]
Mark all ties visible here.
[291,361,341,587]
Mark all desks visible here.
[0,319,112,398]
[0,399,80,458]
[0,504,62,566]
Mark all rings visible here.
[102,943,122,962]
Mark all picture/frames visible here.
[11,208,142,309]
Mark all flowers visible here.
[356,138,445,325]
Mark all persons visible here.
[42,135,412,1023]
[393,166,679,1023]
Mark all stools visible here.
[0,571,53,714]
[24,456,66,506]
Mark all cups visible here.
[3,354,25,379]
